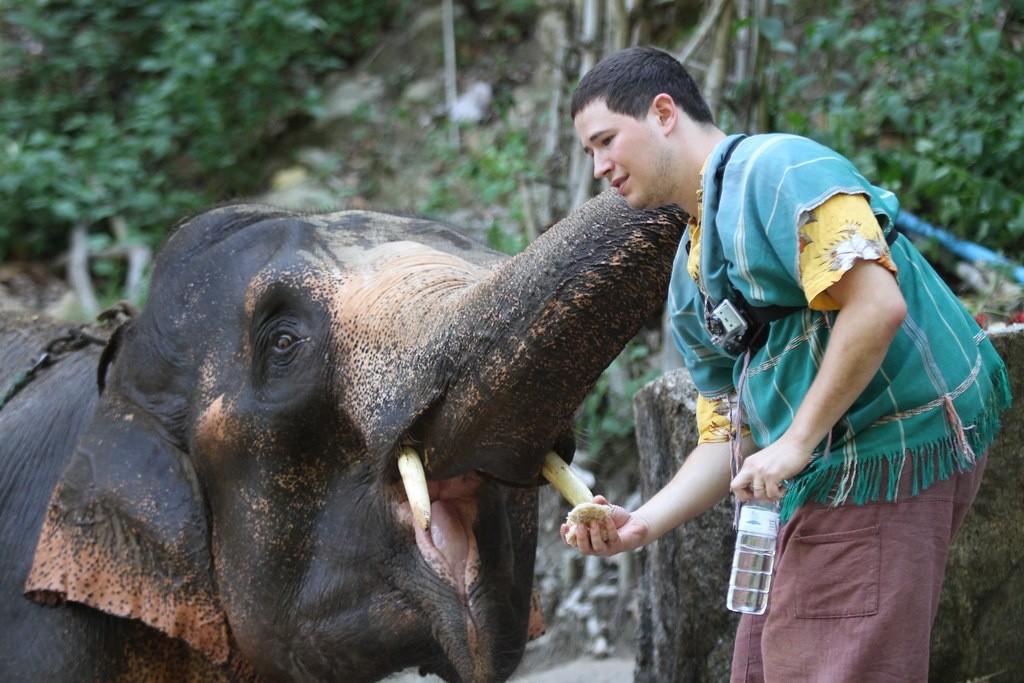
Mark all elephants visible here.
[1,183,691,683]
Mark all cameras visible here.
[704,298,749,348]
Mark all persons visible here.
[558,48,1012,683]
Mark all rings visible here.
[753,485,765,491]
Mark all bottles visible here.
[727,494,781,615]
